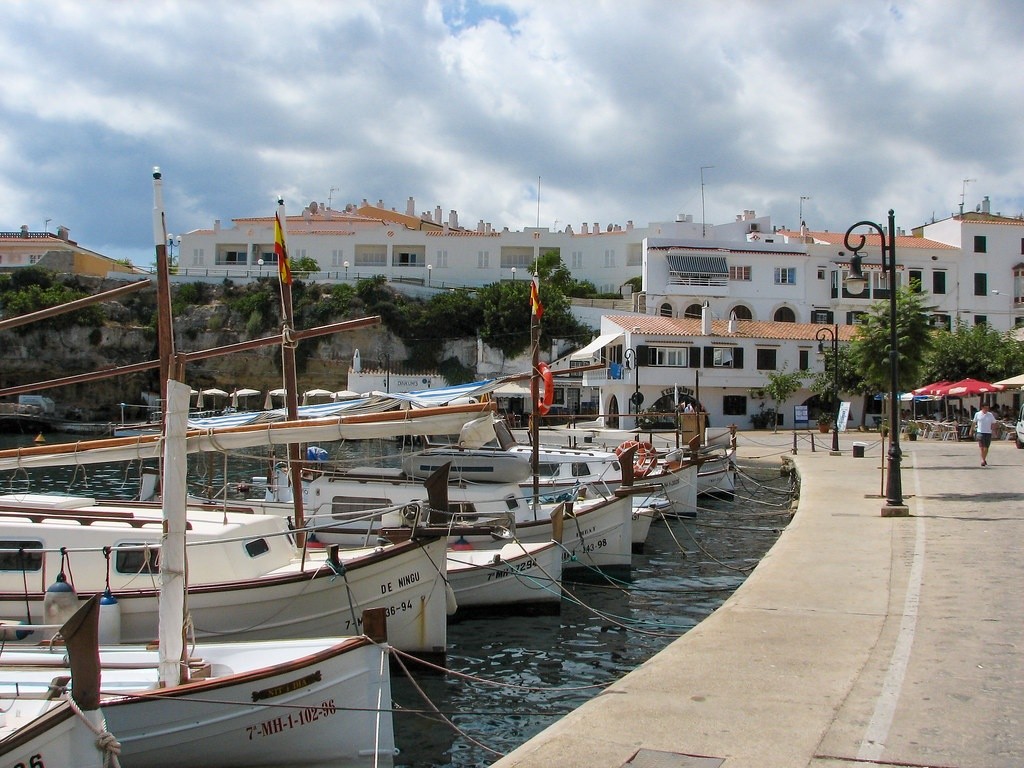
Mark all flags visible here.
[529,280,543,319]
[274,211,292,284]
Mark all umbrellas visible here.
[874,391,944,420]
[931,378,1007,420]
[230,388,261,410]
[190,389,198,395]
[360,391,387,399]
[302,390,309,406]
[493,382,531,398]
[269,389,299,409]
[303,389,333,405]
[202,388,228,410]
[330,390,360,401]
[912,379,959,418]
[232,388,238,412]
[197,388,204,412]
[264,390,273,410]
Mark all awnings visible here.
[571,332,623,360]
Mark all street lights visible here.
[166,233,182,267]
[799,195,810,226]
[45,219,53,232]
[992,289,1012,328]
[426,264,433,288]
[816,323,843,457]
[843,209,910,517]
[343,261,350,280]
[257,258,265,277]
[623,348,641,429]
[510,266,517,281]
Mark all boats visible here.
[0,193,743,604]
[0,166,455,655]
[0,590,125,768]
[0,377,406,768]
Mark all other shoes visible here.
[980,461,987,466]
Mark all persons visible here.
[900,403,1017,424]
[675,401,706,413]
[969,402,999,466]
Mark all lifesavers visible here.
[631,442,658,477]
[530,362,554,415]
[615,440,645,468]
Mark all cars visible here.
[1014,403,1024,449]
